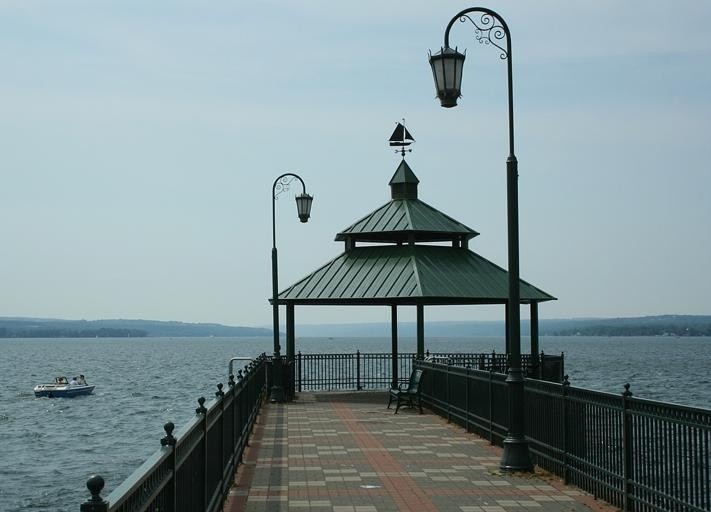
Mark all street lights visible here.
[266,172,316,403]
[428,7,535,476]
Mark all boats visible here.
[31,374,96,401]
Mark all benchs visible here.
[388,368,424,414]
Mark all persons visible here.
[70,377,80,385]
[58,378,64,383]
[79,375,88,386]
